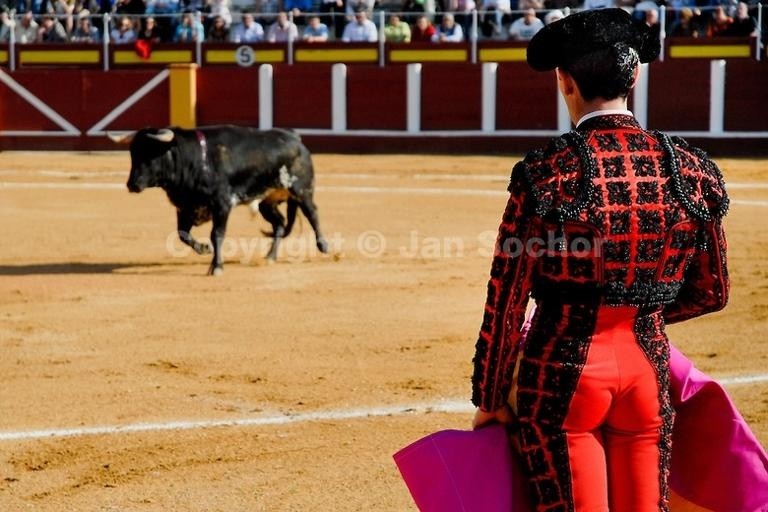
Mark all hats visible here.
[526,8,660,72]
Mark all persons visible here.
[470,3,732,510]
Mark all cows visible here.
[105,122,328,276]
